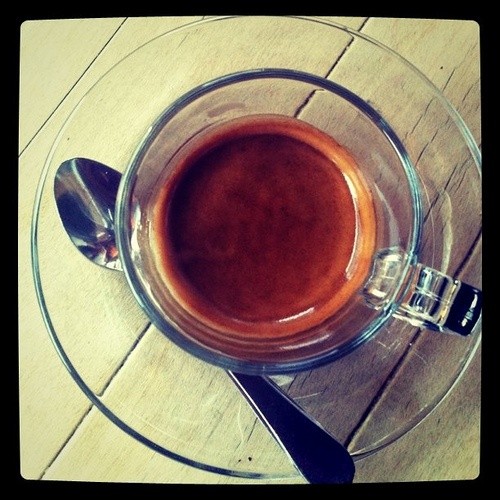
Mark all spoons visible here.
[53,156,356,484]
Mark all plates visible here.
[31,17,482,480]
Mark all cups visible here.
[116,69,481,377]
